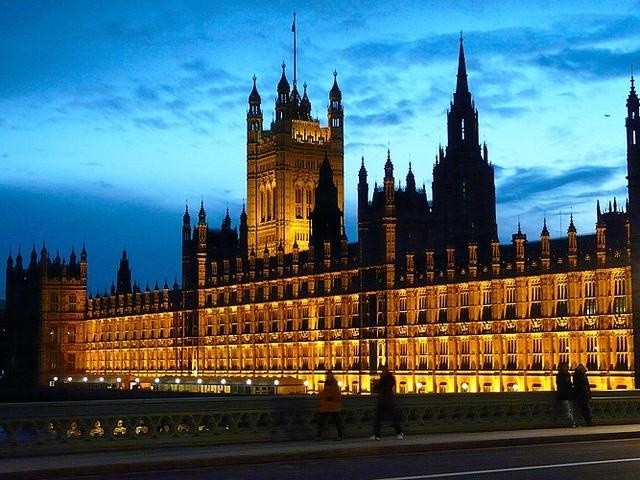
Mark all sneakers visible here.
[313,436,323,441]
[369,435,380,440]
[335,435,341,440]
[397,432,403,439]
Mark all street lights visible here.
[220,379,226,392]
[154,378,160,392]
[135,377,140,390]
[303,380,310,395]
[417,381,423,394]
[274,379,280,394]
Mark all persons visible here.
[367,365,405,442]
[554,361,578,429]
[572,364,596,426]
[313,369,345,442]
